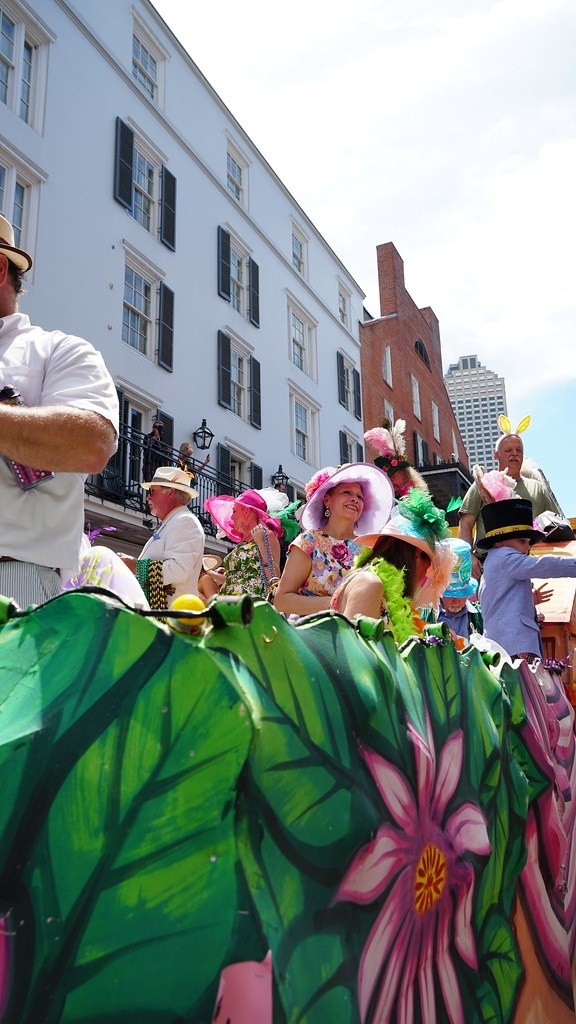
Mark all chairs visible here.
[191,506,215,535]
[98,469,146,510]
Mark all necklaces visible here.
[137,559,168,624]
[255,527,277,598]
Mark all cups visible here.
[0,384,56,492]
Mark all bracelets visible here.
[263,559,273,567]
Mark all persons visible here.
[373,456,428,502]
[204,489,284,600]
[117,467,204,623]
[329,534,456,644]
[0,215,120,607]
[477,538,576,664]
[65,534,151,609]
[198,564,219,600]
[178,443,210,472]
[274,482,364,619]
[457,434,558,585]
[420,598,484,641]
[143,421,170,483]
[274,482,285,493]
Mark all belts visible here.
[0,556,60,576]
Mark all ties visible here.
[153,522,162,534]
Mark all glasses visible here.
[147,488,168,497]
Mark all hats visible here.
[476,499,546,550]
[364,419,409,476]
[352,488,464,577]
[204,489,284,542]
[0,215,32,274]
[441,538,479,599]
[300,462,395,536]
[140,467,200,499]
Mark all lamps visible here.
[271,464,289,486]
[192,419,215,450]
